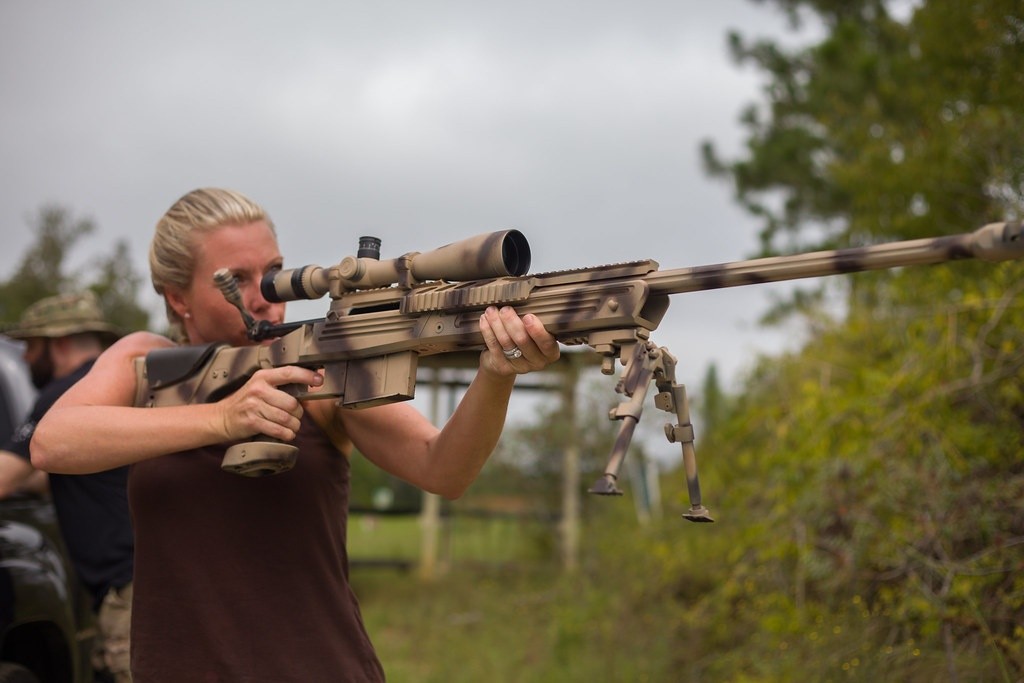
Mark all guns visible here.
[132,229,1024,523]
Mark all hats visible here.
[5,291,126,340]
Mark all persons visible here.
[29,188,560,683]
[0,292,134,683]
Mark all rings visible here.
[504,347,523,361]
